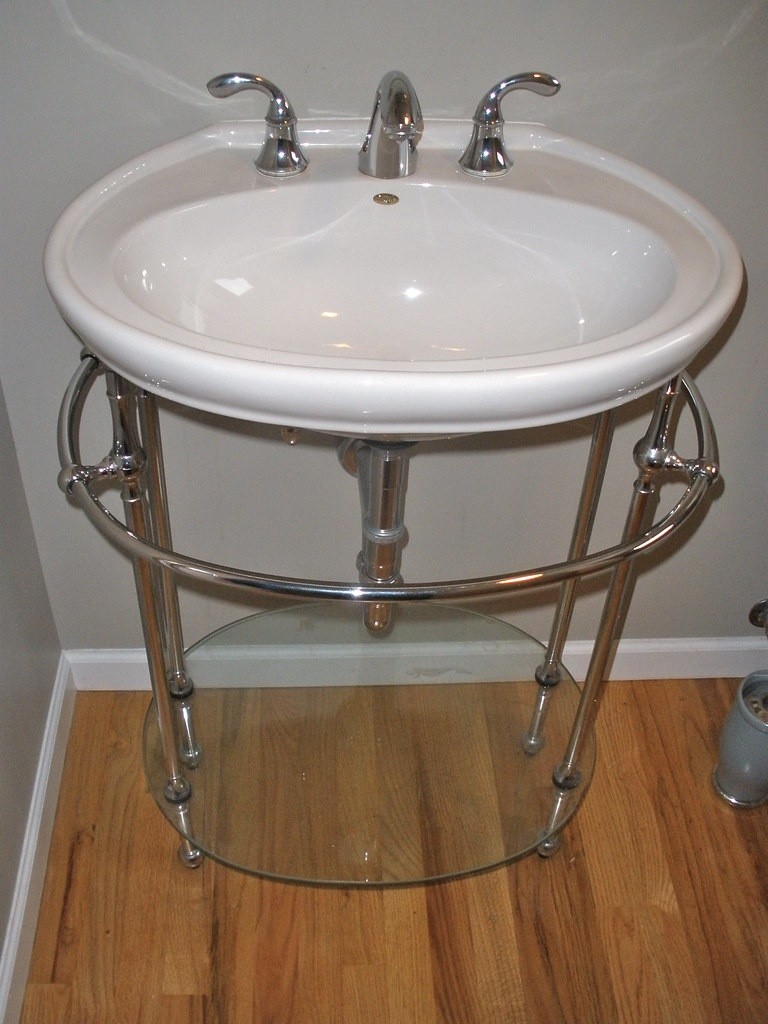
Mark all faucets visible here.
[357,69,425,182]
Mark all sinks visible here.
[39,114,748,444]
